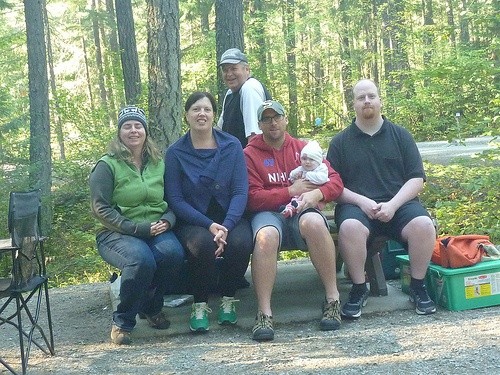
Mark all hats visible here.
[118,105,149,134]
[218,48,249,66]
[300,140,324,166]
[257,99,286,122]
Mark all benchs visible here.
[321,210,388,296]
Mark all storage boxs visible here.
[366,239,500,311]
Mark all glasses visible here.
[259,113,286,124]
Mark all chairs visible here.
[0,189,54,375]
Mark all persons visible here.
[215,47,272,149]
[242,100,344,341]
[164,91,254,332]
[281,140,330,218]
[327,78,436,318]
[89,107,185,343]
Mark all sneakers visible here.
[320,296,343,329]
[141,309,170,330]
[190,300,213,332]
[251,310,275,340]
[111,321,132,345]
[408,283,437,315]
[216,295,241,325]
[341,284,371,318]
